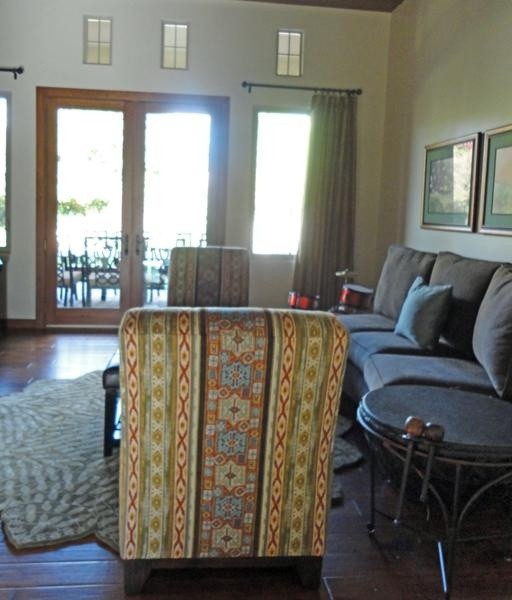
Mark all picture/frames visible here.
[477,124,512,236]
[420,132,482,233]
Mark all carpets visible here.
[0,369,364,559]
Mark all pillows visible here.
[470,263,512,400]
[372,246,436,322]
[394,275,453,353]
[428,251,505,360]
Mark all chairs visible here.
[118,307,350,593]
[56,231,208,308]
[167,247,249,307]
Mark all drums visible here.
[339,283,375,311]
[288,291,321,311]
[328,304,355,315]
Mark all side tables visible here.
[356,385,512,600]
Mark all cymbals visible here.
[335,270,359,278]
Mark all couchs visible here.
[336,246,512,475]
